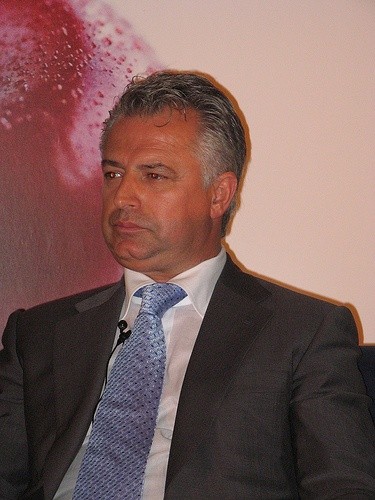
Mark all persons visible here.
[0,71,375,500]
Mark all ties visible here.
[72,281,188,500]
